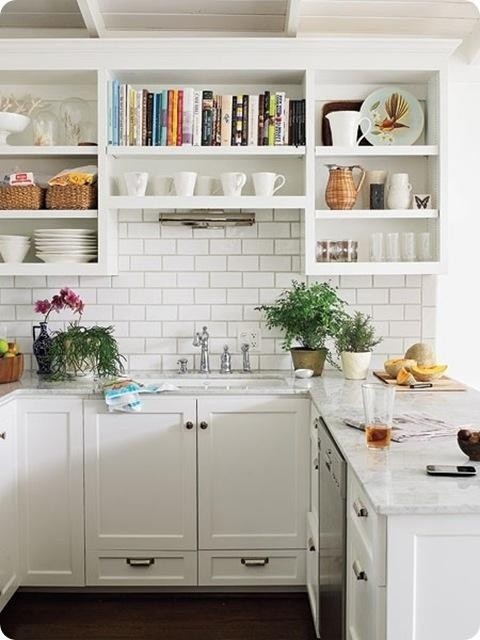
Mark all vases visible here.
[33,322,55,374]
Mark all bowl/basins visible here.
[0,235,31,263]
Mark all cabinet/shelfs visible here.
[0,38,119,274]
[305,37,463,277]
[83,396,311,586]
[18,395,86,586]
[102,38,310,209]
[306,397,319,638]
[0,396,18,610]
[345,463,480,640]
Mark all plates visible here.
[359,88,427,146]
[36,228,98,263]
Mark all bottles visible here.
[386,174,413,209]
[365,170,386,209]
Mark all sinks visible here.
[130,372,290,391]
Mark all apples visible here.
[0,339,20,359]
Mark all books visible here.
[106,79,306,146]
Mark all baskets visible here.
[0,183,45,209]
[0,353,24,383]
[45,184,98,209]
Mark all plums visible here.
[457,430,480,444]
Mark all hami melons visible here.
[384,343,448,386]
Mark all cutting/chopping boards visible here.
[374,371,465,394]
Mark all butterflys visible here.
[415,196,429,209]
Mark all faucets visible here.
[240,344,251,370]
[192,326,211,373]
[219,345,232,374]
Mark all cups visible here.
[114,173,122,193]
[361,384,395,451]
[251,172,285,197]
[219,173,245,199]
[399,234,417,262]
[126,172,148,199]
[174,171,194,194]
[371,234,385,259]
[152,178,174,197]
[418,234,432,262]
[196,177,218,195]
[387,233,404,261]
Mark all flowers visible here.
[33,287,85,322]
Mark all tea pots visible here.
[325,111,371,148]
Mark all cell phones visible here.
[426,465,478,476]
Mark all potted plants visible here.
[46,317,128,383]
[253,279,351,376]
[335,311,383,379]
